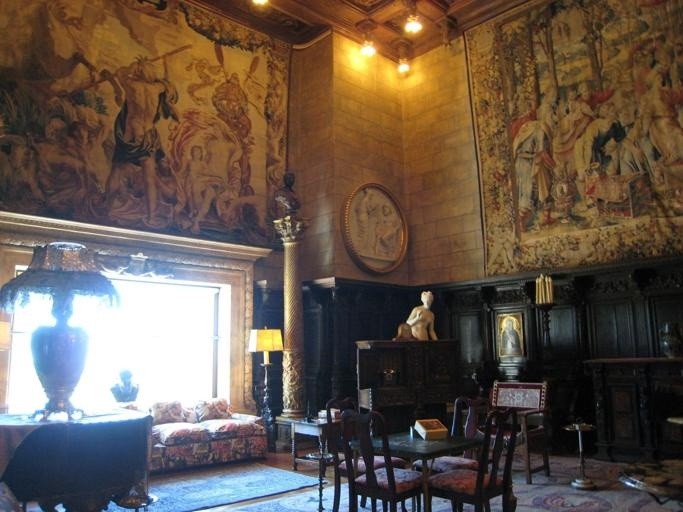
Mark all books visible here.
[318,407,341,420]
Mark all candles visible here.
[535,273,554,304]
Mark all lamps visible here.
[355,0,453,71]
[0,243,120,422]
[248,325,284,453]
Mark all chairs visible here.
[326,378,551,512]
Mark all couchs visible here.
[123,398,268,473]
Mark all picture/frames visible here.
[497,311,525,358]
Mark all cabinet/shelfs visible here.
[584,357,683,463]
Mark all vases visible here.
[659,322,683,359]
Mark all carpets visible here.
[0,458,328,512]
[220,452,683,512]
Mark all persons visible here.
[406,291,438,341]
[376,204,401,254]
[509,63,683,233]
[355,187,378,241]
[273,173,301,219]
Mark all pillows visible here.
[122,398,233,426]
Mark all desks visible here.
[290,417,343,476]
[0,408,153,512]
[616,459,683,505]
